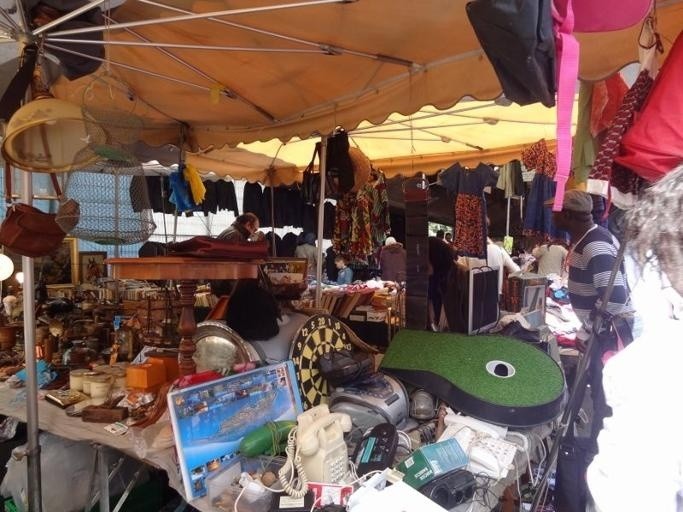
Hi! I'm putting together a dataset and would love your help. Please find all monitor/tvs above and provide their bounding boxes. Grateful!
[466,264,500,337]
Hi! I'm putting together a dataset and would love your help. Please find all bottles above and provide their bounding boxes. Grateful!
[68,364,125,402]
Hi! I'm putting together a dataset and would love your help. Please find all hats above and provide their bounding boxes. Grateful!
[542,189,593,213]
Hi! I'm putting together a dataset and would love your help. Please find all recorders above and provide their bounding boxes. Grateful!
[349,422,399,479]
[328,370,410,443]
[418,467,477,511]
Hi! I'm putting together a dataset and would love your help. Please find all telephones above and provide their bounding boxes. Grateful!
[435,422,518,479]
[295,403,352,483]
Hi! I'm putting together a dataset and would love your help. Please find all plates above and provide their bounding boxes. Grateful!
[175,321,254,373]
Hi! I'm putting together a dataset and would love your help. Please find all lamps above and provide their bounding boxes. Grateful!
[0,33,110,174]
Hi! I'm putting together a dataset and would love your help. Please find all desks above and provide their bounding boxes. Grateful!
[0,343,577,512]
[103,255,263,430]
[337,310,401,348]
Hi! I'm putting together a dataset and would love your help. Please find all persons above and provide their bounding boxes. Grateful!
[445,233,452,243]
[211,213,260,242]
[294,231,326,275]
[334,256,354,286]
[436,230,444,240]
[380,237,407,283]
[532,241,568,276]
[542,190,636,454]
[585,166,683,512]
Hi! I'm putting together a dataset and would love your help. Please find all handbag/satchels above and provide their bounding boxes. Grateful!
[403,187,428,202]
[176,236,273,262]
[0,198,80,257]
[466,0,556,107]
[302,172,343,206]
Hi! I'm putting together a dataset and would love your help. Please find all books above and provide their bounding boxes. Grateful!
[309,289,376,321]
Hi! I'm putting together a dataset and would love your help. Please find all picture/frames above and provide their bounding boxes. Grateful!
[263,255,308,286]
[78,250,107,286]
[0,234,78,291]
